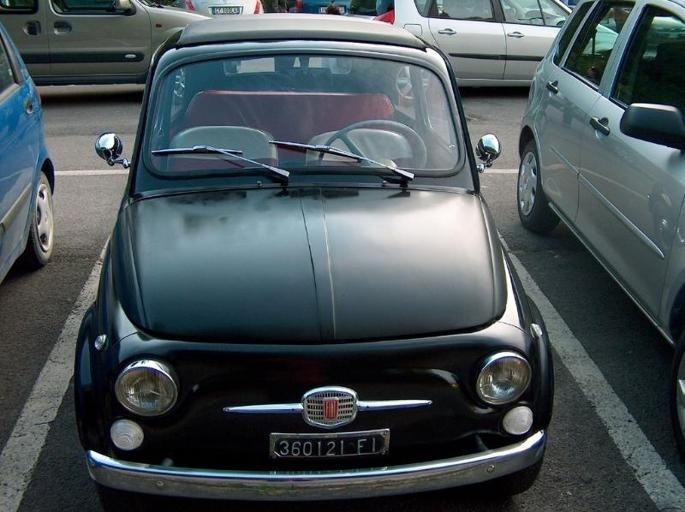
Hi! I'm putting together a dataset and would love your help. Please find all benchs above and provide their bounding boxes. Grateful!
[185,90,395,158]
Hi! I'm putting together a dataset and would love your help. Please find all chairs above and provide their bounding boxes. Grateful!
[306,128,413,168]
[168,126,278,171]
[649,40,685,109]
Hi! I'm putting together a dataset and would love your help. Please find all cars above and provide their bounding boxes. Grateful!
[515,1,685,463]
[0,22,56,286]
[73,14,555,510]
[283,1,352,15]
